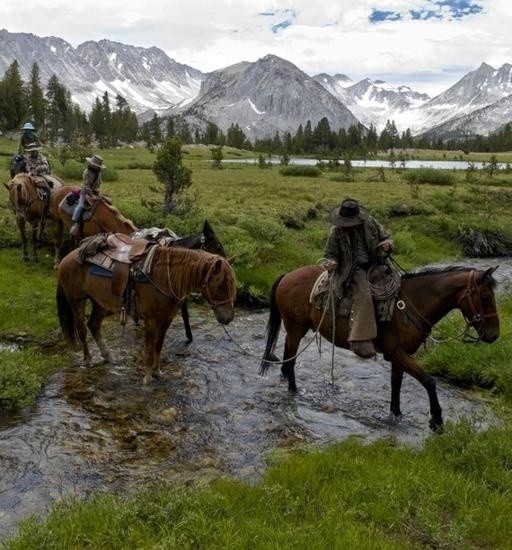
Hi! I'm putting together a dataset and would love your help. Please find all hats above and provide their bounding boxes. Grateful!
[86,155,106,169]
[21,123,35,130]
[24,143,43,152]
[331,198,369,227]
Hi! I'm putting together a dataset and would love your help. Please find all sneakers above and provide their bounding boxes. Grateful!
[69,225,80,236]
[351,340,376,358]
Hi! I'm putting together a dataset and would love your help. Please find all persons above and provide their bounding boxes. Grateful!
[24,142,51,177]
[17,122,44,157]
[322,199,396,358]
[70,154,106,234]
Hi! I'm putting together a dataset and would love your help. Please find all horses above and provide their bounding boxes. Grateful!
[50,183,138,270]
[4,173,65,264]
[129,219,225,344]
[9,151,52,179]
[55,245,238,392]
[259,259,499,438]
